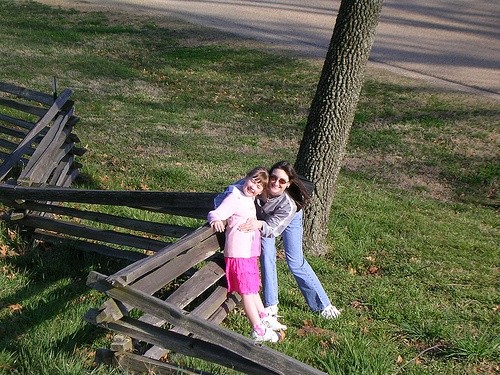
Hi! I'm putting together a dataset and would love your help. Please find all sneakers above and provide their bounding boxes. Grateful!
[252,327,283,343]
[264,304,279,323]
[320,306,342,318]
[260,315,288,331]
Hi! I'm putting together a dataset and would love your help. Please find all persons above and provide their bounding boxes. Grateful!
[208,167,281,342]
[238,161,340,319]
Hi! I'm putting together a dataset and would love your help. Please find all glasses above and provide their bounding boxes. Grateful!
[270,175,289,184]
